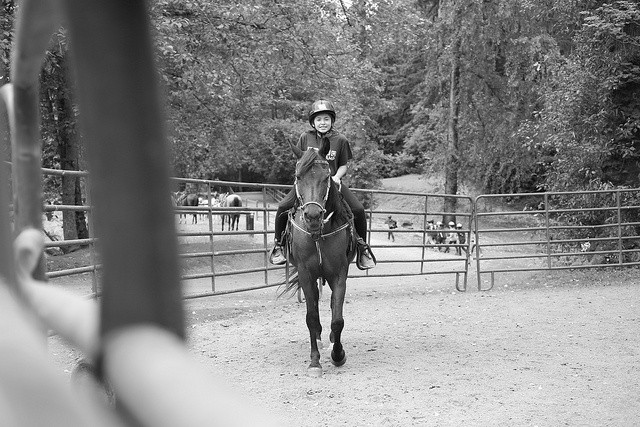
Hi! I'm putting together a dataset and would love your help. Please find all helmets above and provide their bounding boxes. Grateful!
[448,222,454,226]
[457,224,462,228]
[308,100,336,119]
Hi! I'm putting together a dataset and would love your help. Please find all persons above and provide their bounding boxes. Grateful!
[425,219,484,257]
[270,99,377,269]
[385,216,397,241]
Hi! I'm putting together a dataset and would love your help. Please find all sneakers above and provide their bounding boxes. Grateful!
[269,248,287,265]
[360,248,375,268]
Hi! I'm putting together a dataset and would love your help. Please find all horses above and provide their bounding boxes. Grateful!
[173,191,200,224]
[277,135,357,381]
[220,192,243,231]
